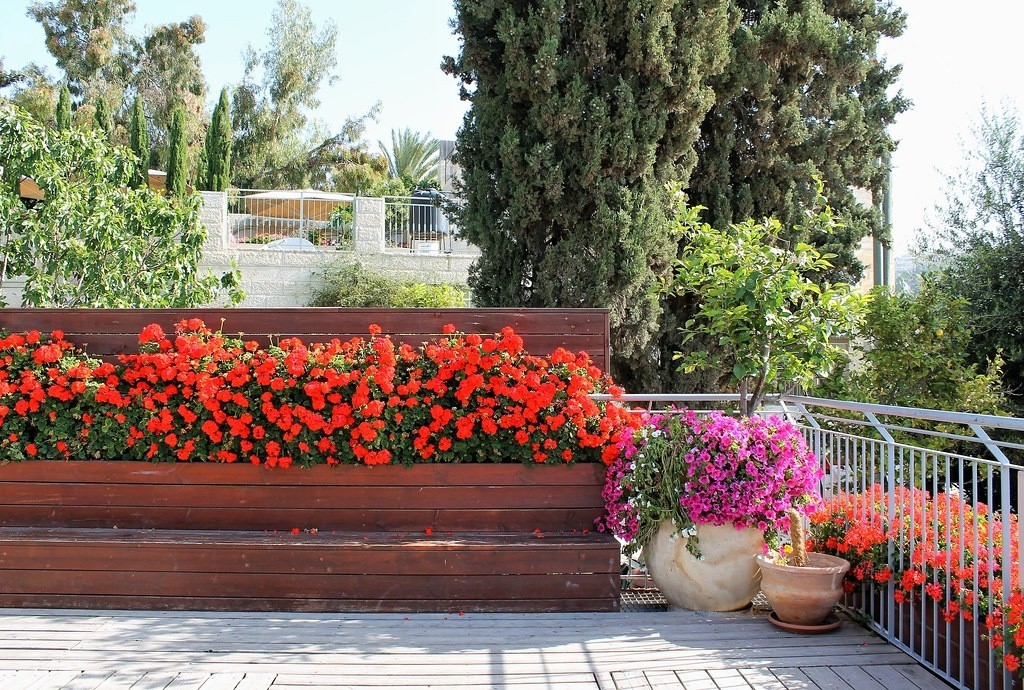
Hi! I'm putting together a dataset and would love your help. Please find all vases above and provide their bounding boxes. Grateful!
[641,517,768,612]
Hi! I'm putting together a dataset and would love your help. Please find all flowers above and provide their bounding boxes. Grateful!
[805,482,1024,690]
[235,233,288,244]
[306,230,336,246]
[0,315,648,466]
[591,403,826,593]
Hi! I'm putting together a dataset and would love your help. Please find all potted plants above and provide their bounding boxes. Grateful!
[756,507,852,624]
